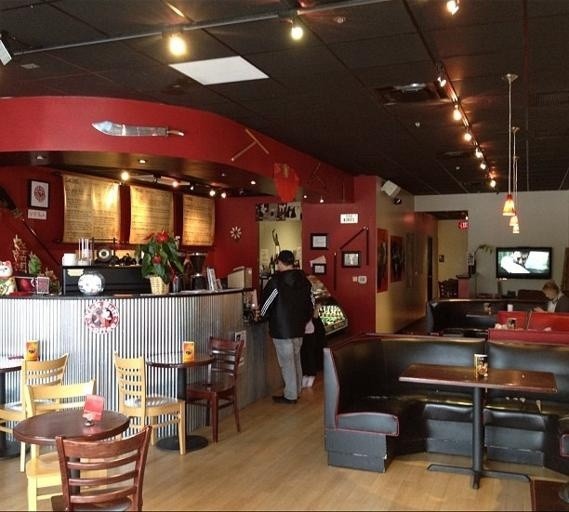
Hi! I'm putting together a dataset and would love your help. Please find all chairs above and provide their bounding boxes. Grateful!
[0,353,69,472]
[186,337,244,444]
[111,351,187,455]
[49,424,153,511]
[25,380,111,511]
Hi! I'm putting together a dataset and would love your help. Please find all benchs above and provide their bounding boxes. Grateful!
[427,290,568,336]
[323,333,568,473]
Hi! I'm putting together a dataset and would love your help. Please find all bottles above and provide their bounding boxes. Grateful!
[78,271,106,297]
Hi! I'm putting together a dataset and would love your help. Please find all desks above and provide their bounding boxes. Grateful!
[398,363,558,487]
[530,479,569,511]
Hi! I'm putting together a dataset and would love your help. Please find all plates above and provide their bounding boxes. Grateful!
[96,246,112,262]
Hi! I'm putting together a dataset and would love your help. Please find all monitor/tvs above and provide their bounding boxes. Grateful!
[495,247,553,279]
[61,265,152,294]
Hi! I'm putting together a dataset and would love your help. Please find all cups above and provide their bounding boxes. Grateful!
[507,304,513,312]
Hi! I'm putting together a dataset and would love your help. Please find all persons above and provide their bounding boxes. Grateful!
[512,249,529,268]
[533,280,569,312]
[260,251,313,403]
[301,295,321,388]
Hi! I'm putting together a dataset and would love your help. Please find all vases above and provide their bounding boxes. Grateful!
[148,276,170,295]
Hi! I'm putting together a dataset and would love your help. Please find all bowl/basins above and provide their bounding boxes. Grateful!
[62,252,89,266]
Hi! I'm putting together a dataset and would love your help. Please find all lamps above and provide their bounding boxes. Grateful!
[500,74,521,233]
[433,63,497,190]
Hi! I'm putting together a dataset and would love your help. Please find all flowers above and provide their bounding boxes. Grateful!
[136,230,185,283]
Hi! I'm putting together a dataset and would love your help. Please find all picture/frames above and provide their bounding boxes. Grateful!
[311,232,327,248]
[313,263,326,274]
[340,251,361,268]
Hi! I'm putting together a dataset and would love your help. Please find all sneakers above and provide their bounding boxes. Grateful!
[271,376,315,404]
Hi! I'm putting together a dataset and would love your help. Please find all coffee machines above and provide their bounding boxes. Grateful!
[183,250,208,290]
